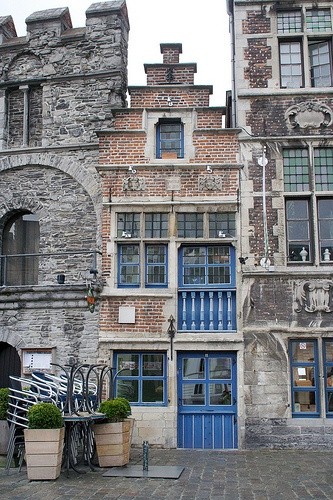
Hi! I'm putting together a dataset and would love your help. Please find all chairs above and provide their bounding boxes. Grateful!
[6,363,131,483]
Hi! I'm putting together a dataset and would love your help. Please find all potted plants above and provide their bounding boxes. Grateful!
[113,398,134,446]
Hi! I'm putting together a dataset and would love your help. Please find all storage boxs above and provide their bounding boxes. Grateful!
[0,388,15,455]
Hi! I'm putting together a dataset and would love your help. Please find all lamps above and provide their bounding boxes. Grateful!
[300,247,308,262]
[323,248,331,261]
